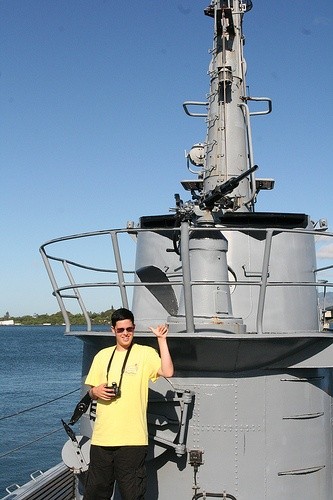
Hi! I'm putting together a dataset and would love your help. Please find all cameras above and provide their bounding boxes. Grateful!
[105,382,121,398]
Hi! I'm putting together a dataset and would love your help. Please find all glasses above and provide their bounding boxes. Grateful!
[115,327,134,333]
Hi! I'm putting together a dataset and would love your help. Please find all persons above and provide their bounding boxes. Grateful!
[83,307,174,500]
